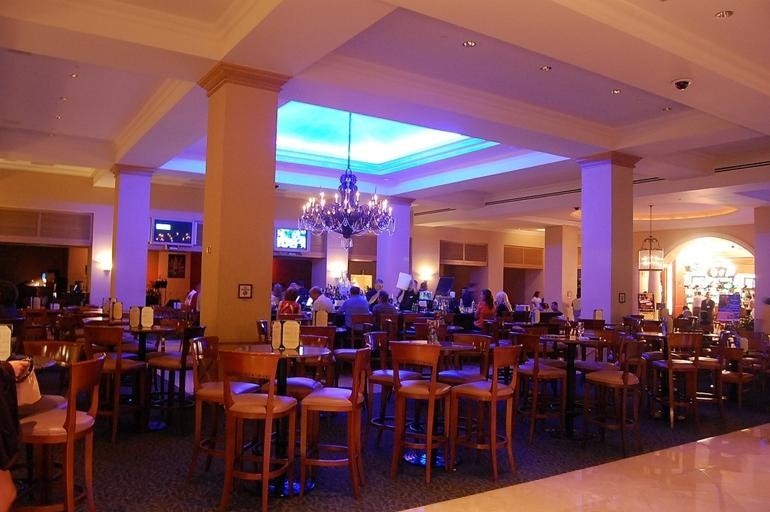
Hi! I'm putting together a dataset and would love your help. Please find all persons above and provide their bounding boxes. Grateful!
[571,293,581,322]
[676,306,691,319]
[700,293,714,312]
[0,281,30,512]
[529,290,565,319]
[395,280,433,311]
[271,279,397,336]
[185,279,200,313]
[447,288,513,335]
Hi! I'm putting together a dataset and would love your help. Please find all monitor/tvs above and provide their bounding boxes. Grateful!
[150,216,195,247]
[273,228,311,252]
[305,296,314,307]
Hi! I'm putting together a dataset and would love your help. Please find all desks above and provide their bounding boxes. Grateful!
[214,343,334,386]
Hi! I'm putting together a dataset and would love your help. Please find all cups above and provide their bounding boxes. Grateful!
[577,322,585,338]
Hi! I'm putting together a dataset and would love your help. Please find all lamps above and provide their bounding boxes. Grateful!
[299,101,396,247]
[637,204,664,272]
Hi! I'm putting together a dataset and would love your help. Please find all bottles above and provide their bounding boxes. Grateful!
[426,321,438,345]
[564,317,571,336]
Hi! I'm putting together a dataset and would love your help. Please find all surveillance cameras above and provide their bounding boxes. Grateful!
[574,207,579,210]
[673,79,691,91]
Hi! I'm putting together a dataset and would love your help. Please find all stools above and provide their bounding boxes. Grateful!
[219,349,298,511]
[272,284,770,443]
[190,335,261,477]
[19,353,108,512]
[298,344,371,498]
[1,284,200,443]
[389,340,455,486]
[450,344,520,484]
[363,331,422,454]
